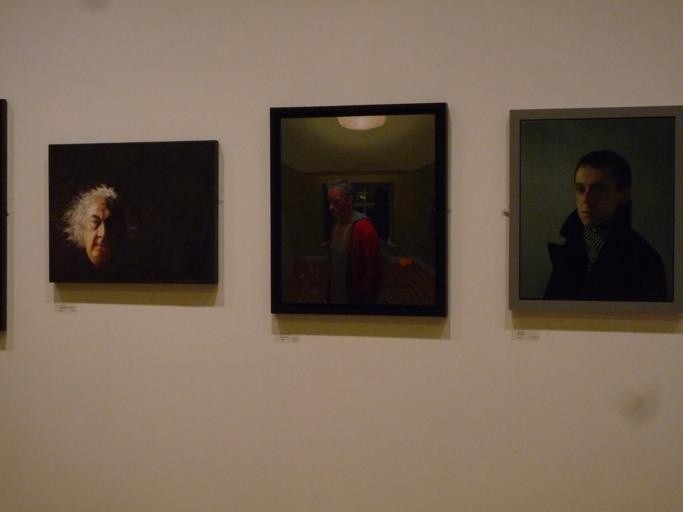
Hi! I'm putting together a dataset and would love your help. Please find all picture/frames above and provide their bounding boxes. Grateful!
[505,103,683,316]
[268,100,451,321]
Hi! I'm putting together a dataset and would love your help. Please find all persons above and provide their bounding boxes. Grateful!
[540,151,667,302]
[51,183,125,281]
[324,177,382,305]
